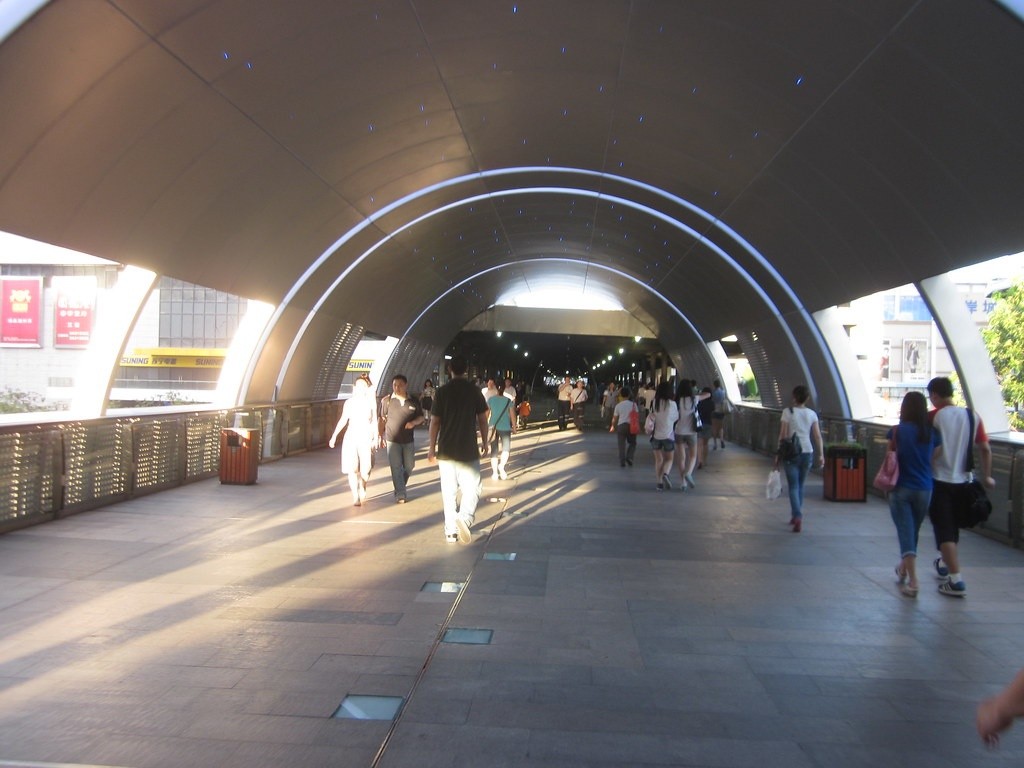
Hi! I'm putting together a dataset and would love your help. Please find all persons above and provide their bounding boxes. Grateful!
[774,385,825,533]
[973,665,1024,750]
[674,379,711,488]
[609,388,639,467]
[427,357,489,544]
[472,378,516,433]
[927,377,996,596]
[557,375,574,430]
[570,380,588,430]
[906,342,920,373]
[885,391,943,597]
[378,374,425,503]
[599,379,657,430]
[418,379,436,426]
[487,380,517,480]
[691,379,725,470]
[516,397,531,430]
[649,381,680,491]
[328,376,379,506]
[881,348,889,378]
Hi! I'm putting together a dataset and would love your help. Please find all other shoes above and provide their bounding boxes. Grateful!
[395,496,408,504]
[490,473,500,480]
[497,464,507,480]
[680,483,688,492]
[790,516,801,532]
[713,445,717,450]
[359,481,367,497]
[685,471,695,488]
[662,473,673,489]
[353,497,362,506]
[721,440,726,449]
[656,483,664,491]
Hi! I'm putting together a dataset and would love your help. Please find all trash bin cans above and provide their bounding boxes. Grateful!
[219,428,261,485]
[823,445,868,502]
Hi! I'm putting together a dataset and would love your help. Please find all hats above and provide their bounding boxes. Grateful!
[360,373,372,387]
[451,352,468,369]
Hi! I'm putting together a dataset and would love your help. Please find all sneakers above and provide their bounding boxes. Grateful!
[901,584,918,598]
[895,565,907,580]
[937,576,967,595]
[933,557,949,580]
[444,525,457,543]
[455,514,471,544]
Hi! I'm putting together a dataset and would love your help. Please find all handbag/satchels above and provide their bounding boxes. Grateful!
[722,399,732,414]
[872,426,900,498]
[950,479,992,528]
[630,402,641,435]
[644,413,656,435]
[766,470,783,500]
[486,425,496,444]
[694,407,704,432]
[774,431,803,460]
